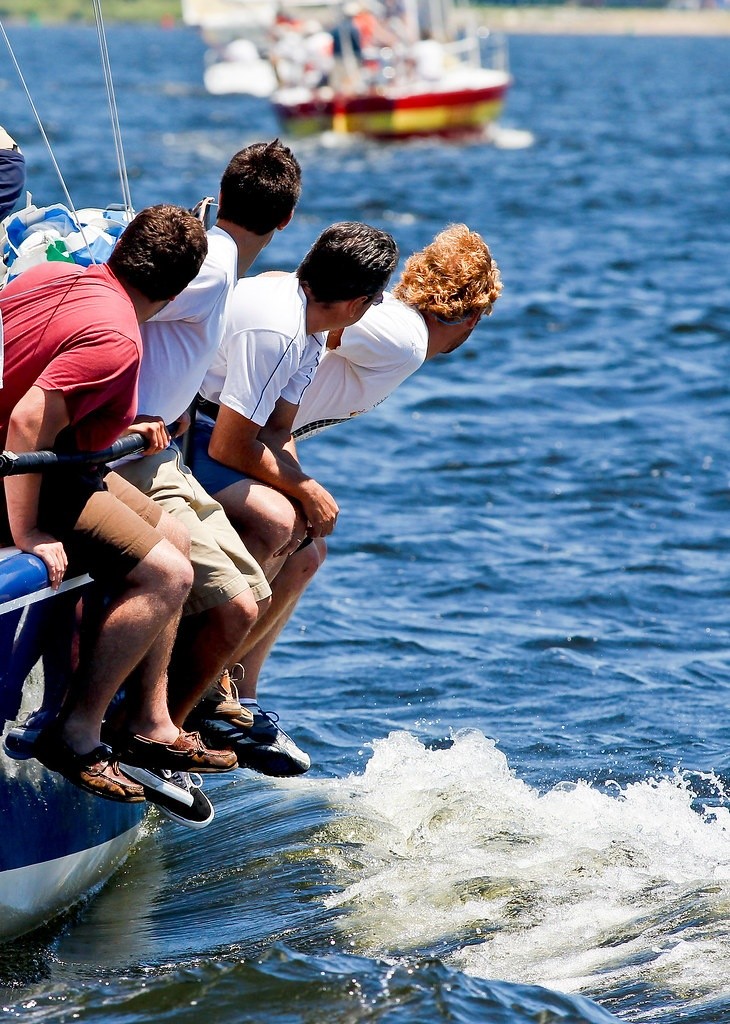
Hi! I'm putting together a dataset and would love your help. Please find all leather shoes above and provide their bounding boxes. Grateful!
[112,725,240,773]
[31,723,147,804]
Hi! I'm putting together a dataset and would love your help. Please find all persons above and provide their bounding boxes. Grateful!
[266,1,445,98]
[0,127,502,807]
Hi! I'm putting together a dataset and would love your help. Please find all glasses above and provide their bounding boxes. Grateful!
[367,293,384,306]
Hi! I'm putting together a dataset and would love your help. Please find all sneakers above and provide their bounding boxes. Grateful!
[194,662,254,727]
[2,706,57,762]
[200,703,311,778]
[117,759,215,829]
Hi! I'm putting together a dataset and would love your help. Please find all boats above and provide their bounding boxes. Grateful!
[0,433,191,948]
[272,0,512,146]
